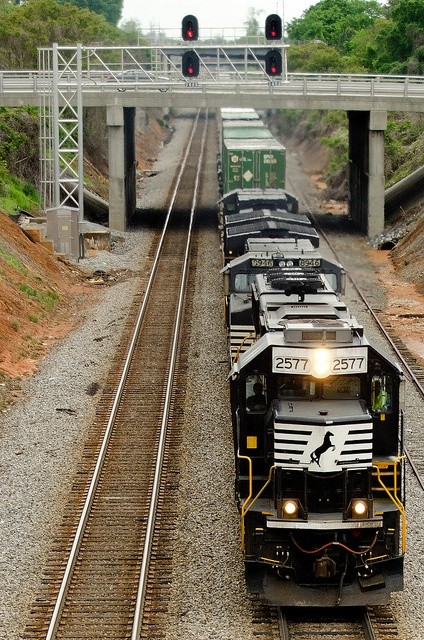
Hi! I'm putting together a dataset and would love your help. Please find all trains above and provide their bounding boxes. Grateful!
[216,106,408,610]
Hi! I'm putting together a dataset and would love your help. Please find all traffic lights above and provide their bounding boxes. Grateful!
[264,50,282,75]
[182,51,199,76]
[181,15,198,41]
[265,14,282,41]
